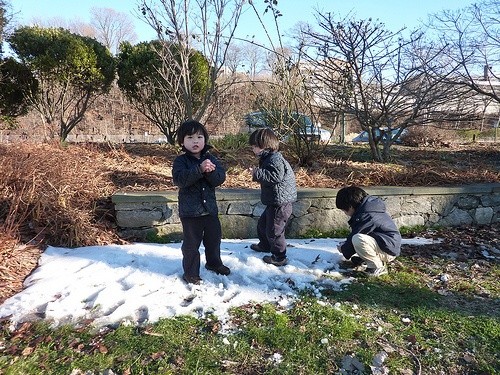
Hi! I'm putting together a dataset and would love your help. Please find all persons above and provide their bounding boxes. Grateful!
[172,121,230,284]
[249,129,297,267]
[335,184,402,278]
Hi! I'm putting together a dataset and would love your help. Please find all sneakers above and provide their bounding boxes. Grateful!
[263,255,287,266]
[351,256,367,268]
[182,272,202,291]
[205,261,230,276]
[363,266,388,277]
[250,244,271,253]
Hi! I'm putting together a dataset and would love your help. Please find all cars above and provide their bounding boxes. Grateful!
[352,126,418,147]
[238,110,331,144]
